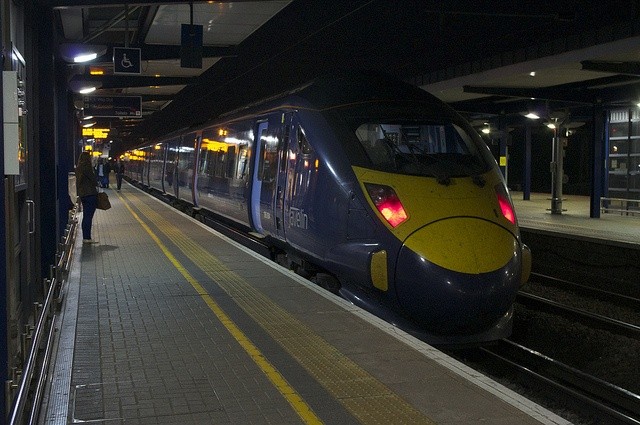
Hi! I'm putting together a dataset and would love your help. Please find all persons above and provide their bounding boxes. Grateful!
[91,155,111,188]
[75,151,101,244]
[114,156,125,193]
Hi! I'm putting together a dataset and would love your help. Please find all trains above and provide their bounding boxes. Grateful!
[113,68,532,345]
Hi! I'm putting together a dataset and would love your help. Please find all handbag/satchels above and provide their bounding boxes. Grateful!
[96,187,111,210]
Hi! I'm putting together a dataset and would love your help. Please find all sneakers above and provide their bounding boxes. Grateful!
[83,239,99,244]
